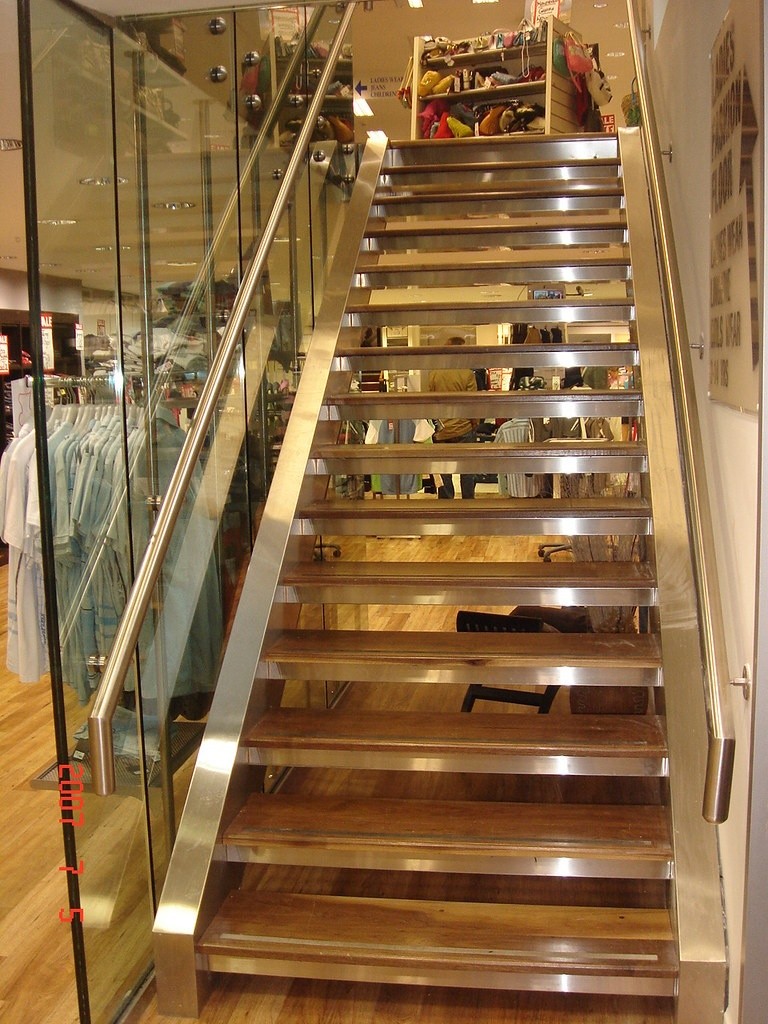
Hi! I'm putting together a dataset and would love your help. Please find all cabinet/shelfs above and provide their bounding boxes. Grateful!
[0,309,82,459]
[255,33,354,147]
[411,14,582,140]
[246,352,307,495]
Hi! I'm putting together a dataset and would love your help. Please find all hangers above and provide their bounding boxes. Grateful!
[4,375,144,456]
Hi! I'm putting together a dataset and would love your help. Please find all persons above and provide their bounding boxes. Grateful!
[428,336,480,499]
